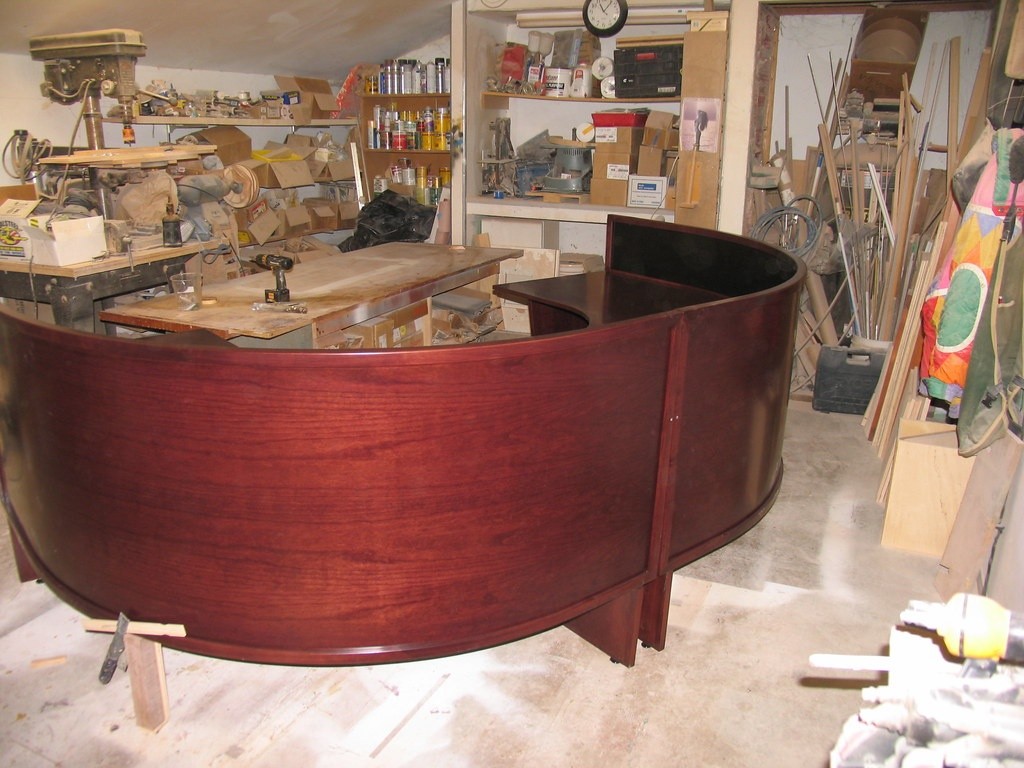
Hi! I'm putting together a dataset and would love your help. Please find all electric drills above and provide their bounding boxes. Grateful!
[250,255,295,303]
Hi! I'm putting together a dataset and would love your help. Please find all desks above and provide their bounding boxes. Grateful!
[99,240,521,355]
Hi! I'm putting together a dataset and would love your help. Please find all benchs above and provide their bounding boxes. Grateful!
[0,214,810,734]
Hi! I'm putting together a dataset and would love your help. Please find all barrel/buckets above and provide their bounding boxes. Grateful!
[545,67,572,98]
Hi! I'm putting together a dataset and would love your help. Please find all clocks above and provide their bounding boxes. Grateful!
[592,56,615,79]
[577,1,627,37]
[600,77,616,99]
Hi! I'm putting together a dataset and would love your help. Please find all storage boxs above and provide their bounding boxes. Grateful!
[0,75,417,276]
[346,296,448,351]
[555,10,728,212]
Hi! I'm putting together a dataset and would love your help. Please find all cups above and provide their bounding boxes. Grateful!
[170,273,203,310]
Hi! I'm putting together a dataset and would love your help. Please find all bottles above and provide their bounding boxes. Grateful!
[363,58,451,205]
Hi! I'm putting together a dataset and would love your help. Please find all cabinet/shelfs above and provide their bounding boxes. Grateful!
[356,91,450,208]
[448,0,780,265]
